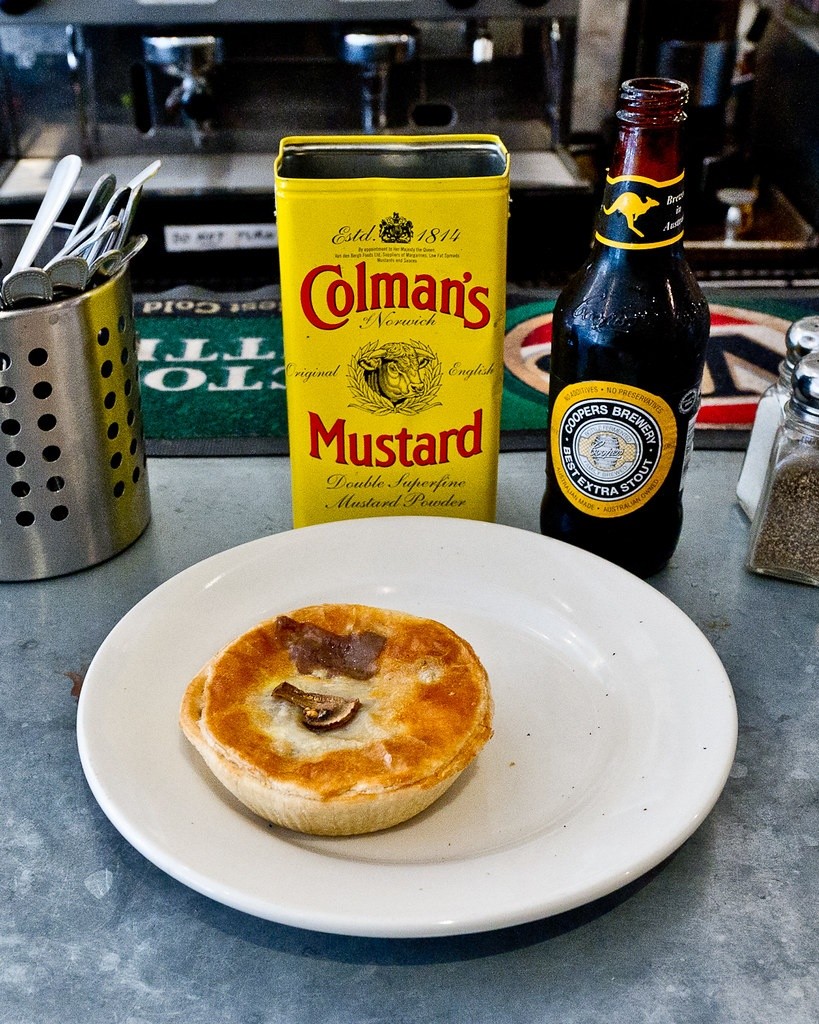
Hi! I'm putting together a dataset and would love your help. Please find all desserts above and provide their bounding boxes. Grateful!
[178,603,497,837]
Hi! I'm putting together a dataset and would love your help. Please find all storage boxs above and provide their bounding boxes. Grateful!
[274,134,513,529]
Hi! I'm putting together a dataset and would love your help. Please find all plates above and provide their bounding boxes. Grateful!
[74,516,741,939]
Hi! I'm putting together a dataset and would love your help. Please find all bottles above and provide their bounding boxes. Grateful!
[737,316,819,521]
[745,349,819,587]
[539,77,711,578]
